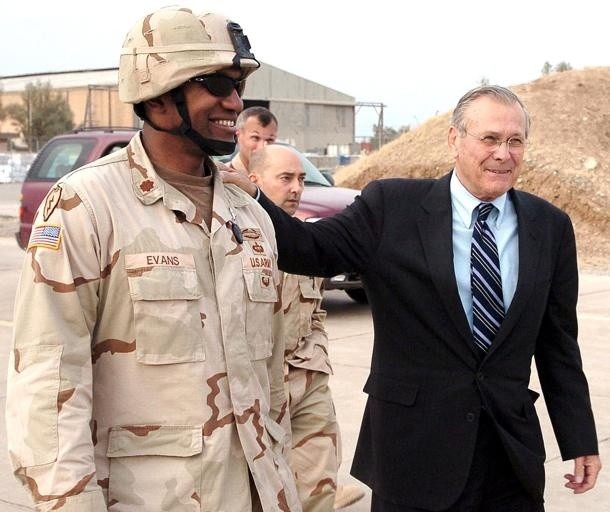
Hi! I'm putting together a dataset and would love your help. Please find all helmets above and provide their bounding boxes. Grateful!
[118,5,261,104]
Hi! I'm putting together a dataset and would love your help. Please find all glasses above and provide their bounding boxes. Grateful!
[189,70,247,98]
[465,129,525,149]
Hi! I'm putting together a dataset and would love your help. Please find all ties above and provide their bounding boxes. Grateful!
[470,202,506,353]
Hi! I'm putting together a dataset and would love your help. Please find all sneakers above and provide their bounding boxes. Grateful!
[332,483,368,512]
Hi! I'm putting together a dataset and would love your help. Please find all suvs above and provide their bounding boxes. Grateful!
[17,123,386,305]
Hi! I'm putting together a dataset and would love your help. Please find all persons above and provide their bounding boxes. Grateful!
[213,84,603,511]
[248,143,371,511]
[8,8,346,512]
[324,141,351,167]
[224,106,279,182]
